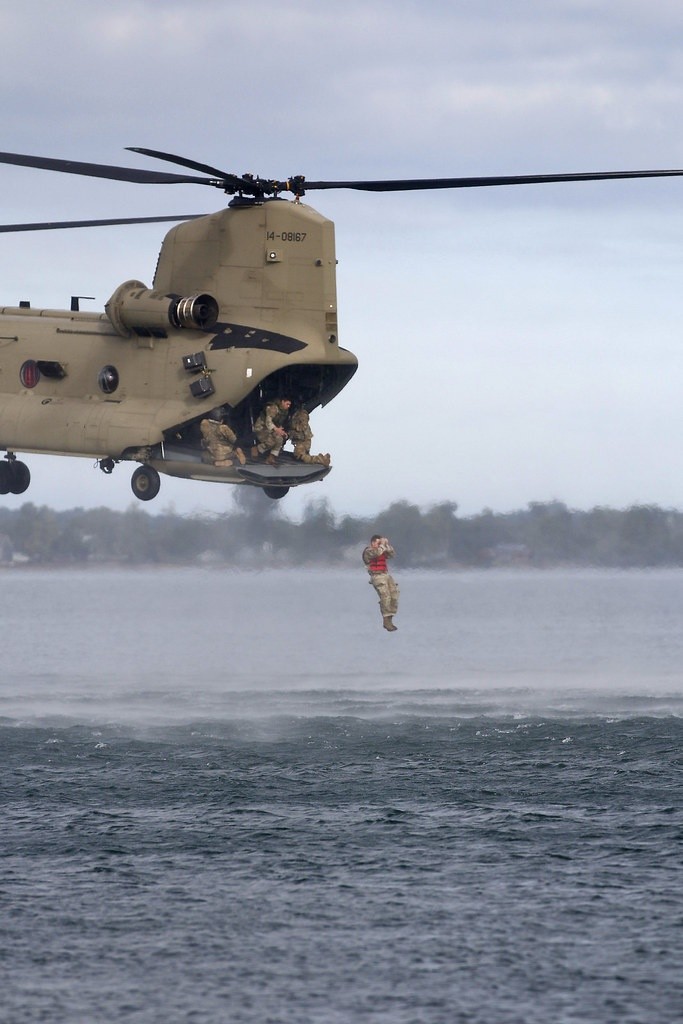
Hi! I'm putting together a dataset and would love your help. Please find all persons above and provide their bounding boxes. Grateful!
[362,535,400,633]
[201,395,329,465]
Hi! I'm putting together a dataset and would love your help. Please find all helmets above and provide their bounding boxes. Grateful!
[209,407,231,422]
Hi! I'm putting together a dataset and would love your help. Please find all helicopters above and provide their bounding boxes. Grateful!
[0,143,682,502]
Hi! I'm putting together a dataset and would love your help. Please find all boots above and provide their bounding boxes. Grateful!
[264,453,281,467]
[383,615,397,632]
[214,459,234,467]
[318,453,330,468]
[250,445,260,463]
[235,447,246,465]
